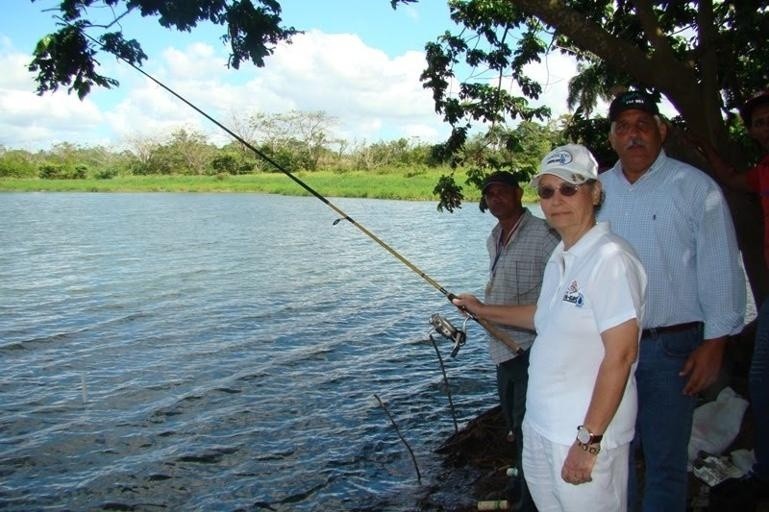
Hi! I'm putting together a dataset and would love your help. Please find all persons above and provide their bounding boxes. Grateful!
[450,143,651,512]
[480,170,562,511]
[594,91,749,511]
[676,90,769,511]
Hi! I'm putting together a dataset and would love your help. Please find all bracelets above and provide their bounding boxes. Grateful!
[575,438,602,455]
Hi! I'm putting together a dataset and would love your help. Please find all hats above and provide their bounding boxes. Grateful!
[535,145,599,186]
[609,91,658,120]
[480,170,517,193]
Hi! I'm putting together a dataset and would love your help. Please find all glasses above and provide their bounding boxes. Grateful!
[537,182,587,199]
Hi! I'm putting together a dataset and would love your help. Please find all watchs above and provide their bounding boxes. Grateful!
[576,424,604,446]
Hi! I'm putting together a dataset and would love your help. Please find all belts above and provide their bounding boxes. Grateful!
[644,323,700,338]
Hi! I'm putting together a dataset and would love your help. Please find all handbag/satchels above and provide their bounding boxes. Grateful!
[689,388,748,471]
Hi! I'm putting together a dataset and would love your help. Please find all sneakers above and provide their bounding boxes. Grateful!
[693,449,741,486]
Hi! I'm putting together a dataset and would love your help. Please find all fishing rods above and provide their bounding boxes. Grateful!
[48,14,525,357]
